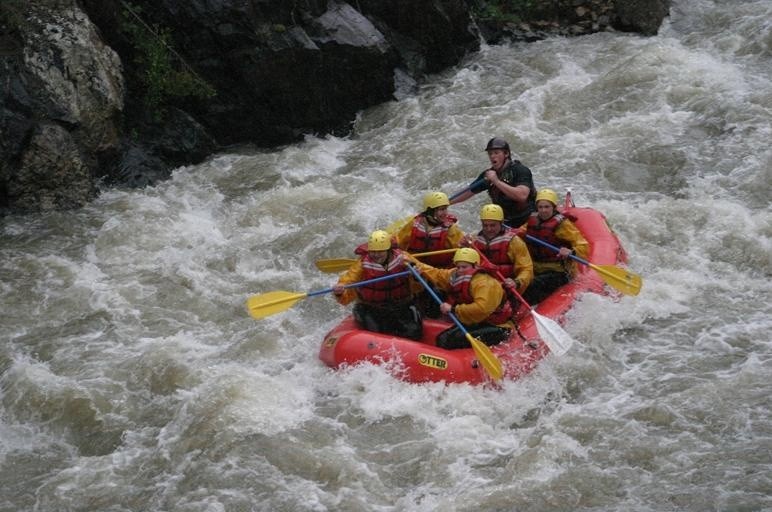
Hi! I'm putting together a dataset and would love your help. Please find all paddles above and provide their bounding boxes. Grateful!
[315,247,460,273]
[248,269,411,320]
[501,222,642,297]
[467,237,573,357]
[373,171,492,238]
[402,261,505,381]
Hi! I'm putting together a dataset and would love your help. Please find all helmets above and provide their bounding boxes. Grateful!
[453,247,480,264]
[534,189,560,206]
[485,137,509,150]
[422,193,449,212]
[479,203,503,219]
[367,229,393,253]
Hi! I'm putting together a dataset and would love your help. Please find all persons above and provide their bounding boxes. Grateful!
[468,204,533,320]
[401,248,514,350]
[446,137,537,242]
[333,230,422,342]
[395,192,467,318]
[521,188,590,308]
